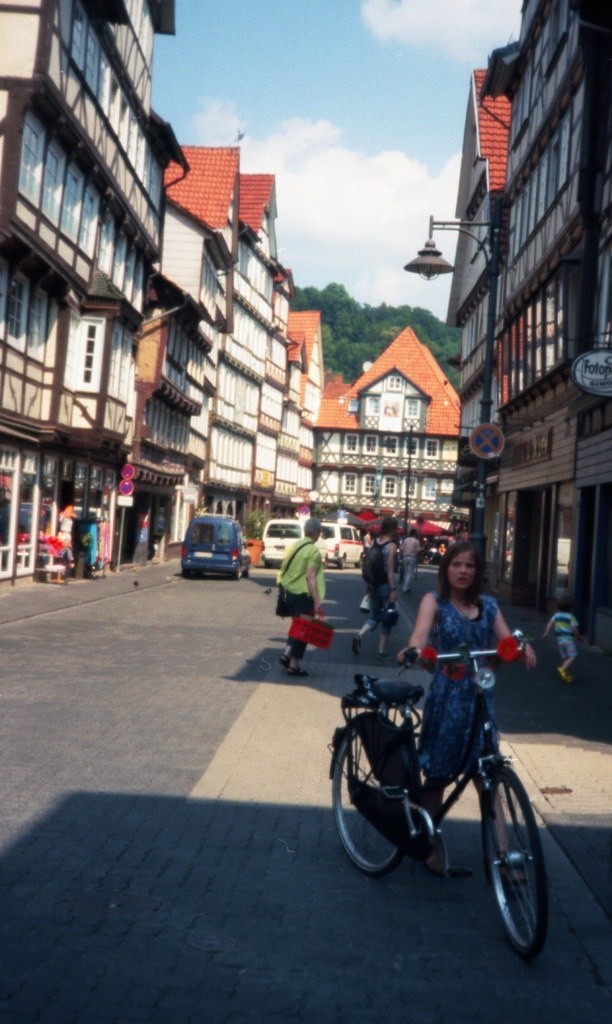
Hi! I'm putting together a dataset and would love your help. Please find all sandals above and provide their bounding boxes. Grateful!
[279,655,289,667]
[288,666,308,676]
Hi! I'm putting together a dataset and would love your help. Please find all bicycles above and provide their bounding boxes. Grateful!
[324,627,548,964]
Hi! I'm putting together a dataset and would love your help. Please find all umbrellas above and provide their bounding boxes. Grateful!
[348,513,453,537]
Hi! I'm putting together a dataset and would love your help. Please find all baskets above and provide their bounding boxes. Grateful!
[288,610,336,651]
[381,600,400,627]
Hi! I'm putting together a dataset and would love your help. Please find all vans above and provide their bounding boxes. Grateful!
[180,516,253,580]
[321,523,367,568]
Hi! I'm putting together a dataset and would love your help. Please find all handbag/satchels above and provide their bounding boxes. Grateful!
[275,587,291,617]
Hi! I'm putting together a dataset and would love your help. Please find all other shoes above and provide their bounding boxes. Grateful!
[378,652,394,659]
[352,635,360,656]
[498,864,528,886]
[566,675,574,682]
[558,667,567,679]
[403,588,411,593]
[422,854,450,880]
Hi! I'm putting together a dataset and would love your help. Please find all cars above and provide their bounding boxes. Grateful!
[260,520,306,568]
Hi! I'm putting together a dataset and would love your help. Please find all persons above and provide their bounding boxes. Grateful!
[542,594,584,684]
[57,503,78,569]
[351,517,399,659]
[362,530,456,592]
[395,540,538,889]
[275,517,326,677]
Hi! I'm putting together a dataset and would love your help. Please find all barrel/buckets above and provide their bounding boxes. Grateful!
[380,600,400,625]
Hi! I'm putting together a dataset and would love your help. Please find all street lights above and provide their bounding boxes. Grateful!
[405,215,505,595]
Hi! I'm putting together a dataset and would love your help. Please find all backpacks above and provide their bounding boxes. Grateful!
[362,535,399,582]
